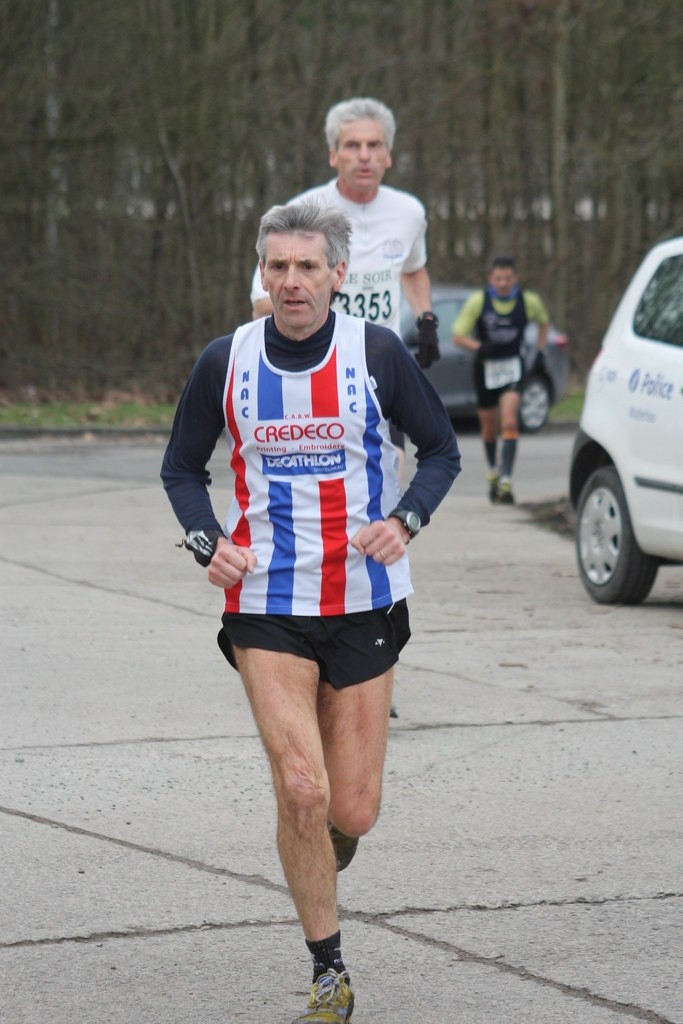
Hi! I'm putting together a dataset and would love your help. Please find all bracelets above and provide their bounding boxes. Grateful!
[422,312,438,321]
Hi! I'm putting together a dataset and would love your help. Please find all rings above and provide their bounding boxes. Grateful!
[379,550,386,558]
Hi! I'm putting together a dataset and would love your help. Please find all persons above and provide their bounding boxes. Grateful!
[160,205,461,1024]
[250,98,446,507]
[451,256,549,503]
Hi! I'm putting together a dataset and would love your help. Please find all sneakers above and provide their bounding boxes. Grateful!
[328,821,360,872]
[291,968,354,1024]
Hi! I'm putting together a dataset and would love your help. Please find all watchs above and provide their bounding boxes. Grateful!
[389,508,422,538]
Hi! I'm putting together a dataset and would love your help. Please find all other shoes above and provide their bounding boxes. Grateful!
[488,469,512,502]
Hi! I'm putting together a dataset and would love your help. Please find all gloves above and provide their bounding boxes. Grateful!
[411,313,441,368]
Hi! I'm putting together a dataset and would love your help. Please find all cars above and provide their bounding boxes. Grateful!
[400,285,569,434]
[569,239,683,605]
